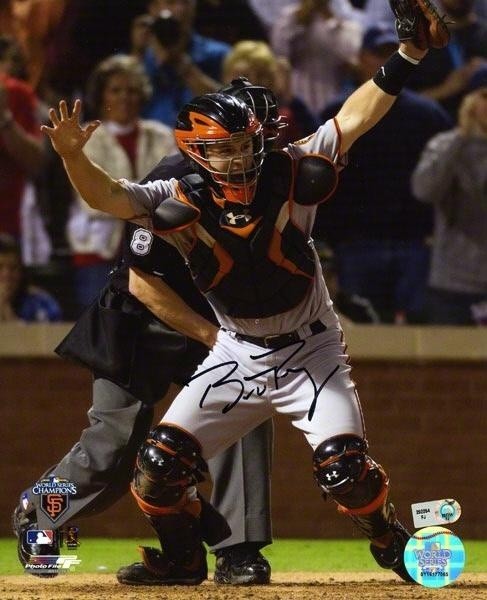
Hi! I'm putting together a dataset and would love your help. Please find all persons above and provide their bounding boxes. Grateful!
[11,76,291,584]
[39,0,453,585]
[0,0,487,326]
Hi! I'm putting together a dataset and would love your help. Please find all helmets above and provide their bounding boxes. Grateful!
[173,76,288,207]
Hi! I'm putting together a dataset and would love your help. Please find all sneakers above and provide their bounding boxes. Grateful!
[116,544,208,587]
[213,548,270,587]
[370,504,415,582]
[11,505,61,578]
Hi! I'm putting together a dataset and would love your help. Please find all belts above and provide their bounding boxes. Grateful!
[223,319,328,350]
